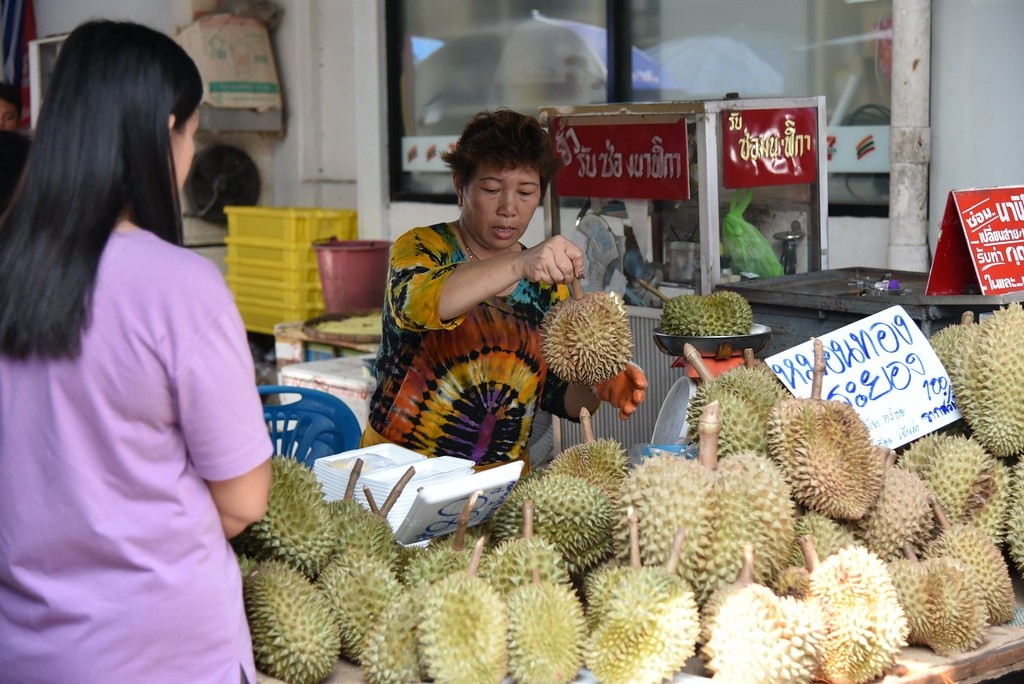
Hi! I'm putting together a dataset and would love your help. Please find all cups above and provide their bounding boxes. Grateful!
[669,242,700,283]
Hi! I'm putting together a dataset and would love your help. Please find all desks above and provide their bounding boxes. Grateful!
[272,323,380,357]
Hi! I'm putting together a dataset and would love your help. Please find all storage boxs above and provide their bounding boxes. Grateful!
[220,206,358,337]
[277,352,377,434]
[174,13,282,115]
[312,442,526,547]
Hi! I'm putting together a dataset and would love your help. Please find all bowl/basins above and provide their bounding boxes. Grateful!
[314,442,525,552]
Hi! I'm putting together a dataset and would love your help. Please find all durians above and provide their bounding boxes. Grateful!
[228,289,1024,684]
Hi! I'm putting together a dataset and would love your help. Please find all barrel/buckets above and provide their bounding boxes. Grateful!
[314,235,393,314]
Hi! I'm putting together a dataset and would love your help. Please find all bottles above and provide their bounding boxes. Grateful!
[623,224,645,281]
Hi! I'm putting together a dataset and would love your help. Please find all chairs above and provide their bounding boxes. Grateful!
[258,384,361,470]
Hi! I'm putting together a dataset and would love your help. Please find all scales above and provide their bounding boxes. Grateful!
[649,324,773,446]
